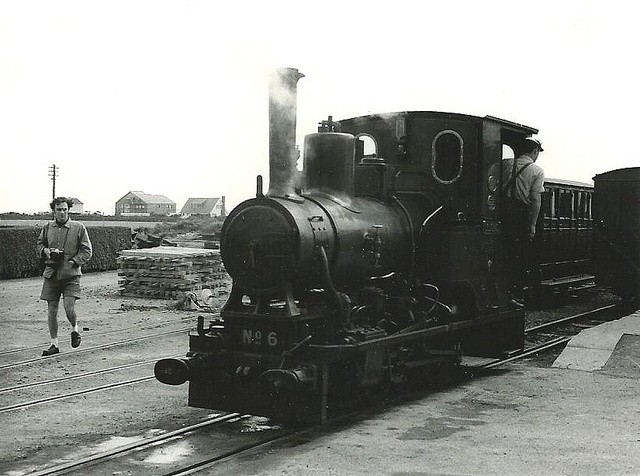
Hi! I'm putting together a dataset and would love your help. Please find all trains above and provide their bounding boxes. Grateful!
[154,68,539,425]
[592,167,640,309]
[523,177,594,279]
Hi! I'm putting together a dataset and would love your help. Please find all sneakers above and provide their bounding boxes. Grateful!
[506,298,524,309]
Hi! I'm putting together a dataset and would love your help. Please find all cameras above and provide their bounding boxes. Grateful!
[50,250,65,260]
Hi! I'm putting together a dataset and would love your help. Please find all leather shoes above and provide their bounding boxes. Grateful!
[43,345,59,355]
[71,332,81,348]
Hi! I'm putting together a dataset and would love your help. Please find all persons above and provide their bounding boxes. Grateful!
[35,196,93,356]
[501,134,544,293]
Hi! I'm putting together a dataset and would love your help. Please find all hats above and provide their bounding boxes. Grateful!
[525,135,543,152]
